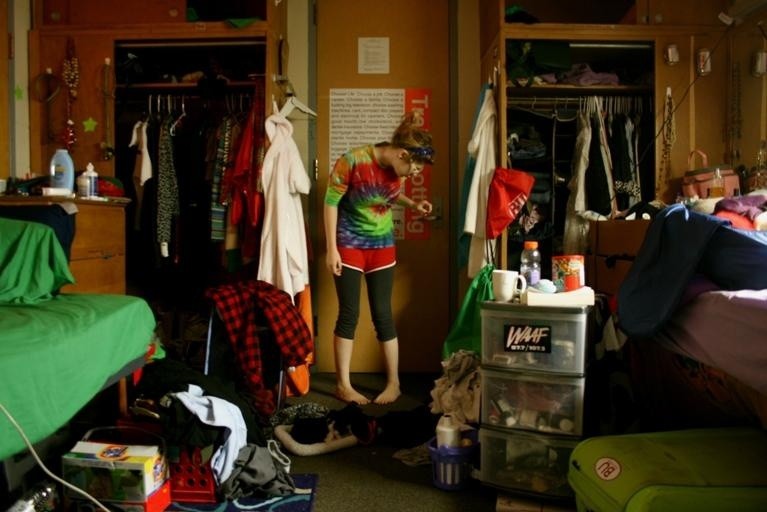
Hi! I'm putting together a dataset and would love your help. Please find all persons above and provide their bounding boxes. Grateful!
[322,110,434,406]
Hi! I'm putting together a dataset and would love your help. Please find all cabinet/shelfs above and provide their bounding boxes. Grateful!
[475,300,589,505]
[2,197,130,417]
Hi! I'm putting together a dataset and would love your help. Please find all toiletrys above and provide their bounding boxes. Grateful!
[50,148,75,192]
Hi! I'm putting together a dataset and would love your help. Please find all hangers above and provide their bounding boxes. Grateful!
[277,80,320,122]
[168,94,205,137]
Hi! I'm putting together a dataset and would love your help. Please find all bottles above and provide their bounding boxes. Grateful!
[82,163,98,197]
[709,168,725,197]
[521,241,541,283]
[490,392,515,430]
[549,412,573,430]
[7,485,50,511]
[435,415,460,486]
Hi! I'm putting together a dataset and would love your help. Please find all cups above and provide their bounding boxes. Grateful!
[492,270,526,303]
[563,273,581,290]
